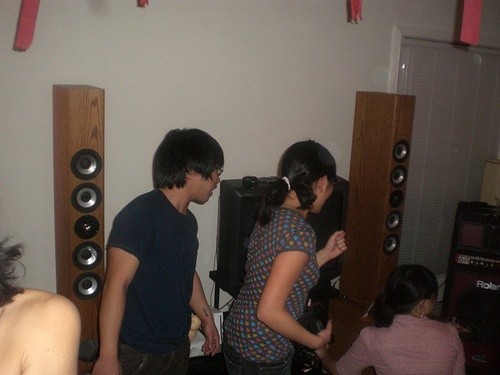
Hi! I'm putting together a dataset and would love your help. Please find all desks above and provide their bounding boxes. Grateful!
[208,271,342,375]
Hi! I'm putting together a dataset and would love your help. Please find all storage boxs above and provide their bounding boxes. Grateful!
[479,158,500,208]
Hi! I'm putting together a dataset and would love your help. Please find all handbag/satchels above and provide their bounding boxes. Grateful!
[289,308,336,350]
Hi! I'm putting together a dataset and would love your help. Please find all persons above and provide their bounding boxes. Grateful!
[0,235,81,375]
[222,140,348,375]
[316,264,465,374]
[92,128,224,375]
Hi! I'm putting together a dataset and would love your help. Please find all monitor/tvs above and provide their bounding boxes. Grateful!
[216,174,350,282]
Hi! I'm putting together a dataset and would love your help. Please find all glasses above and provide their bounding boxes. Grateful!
[213,166,224,177]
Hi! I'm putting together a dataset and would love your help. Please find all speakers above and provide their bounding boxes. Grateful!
[341,90,416,311]
[53,85,106,375]
[440,200,500,375]
